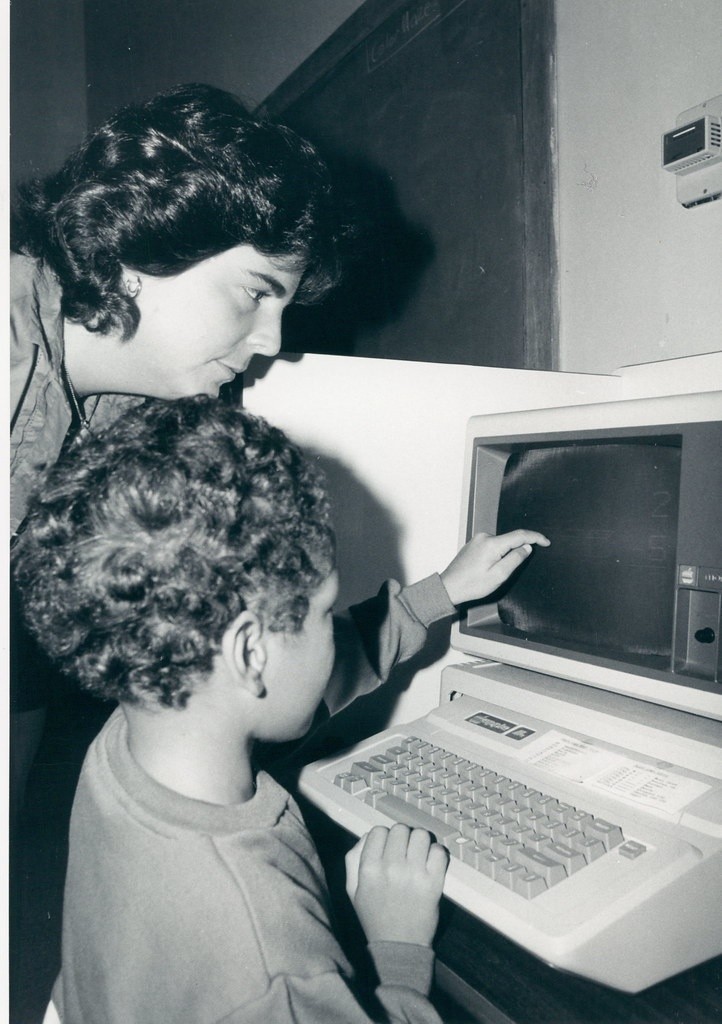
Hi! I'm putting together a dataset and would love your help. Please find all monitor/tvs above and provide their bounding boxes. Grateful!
[449,391,721,722]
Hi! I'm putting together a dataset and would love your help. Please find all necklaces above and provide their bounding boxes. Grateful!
[67,374,101,428]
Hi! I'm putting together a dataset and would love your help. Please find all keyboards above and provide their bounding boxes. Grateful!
[299,724,700,935]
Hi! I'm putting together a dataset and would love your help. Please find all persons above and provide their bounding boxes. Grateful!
[10,87,368,814]
[10,391,550,1024]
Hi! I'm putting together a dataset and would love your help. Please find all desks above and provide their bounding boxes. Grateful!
[329,834,722,1024]
